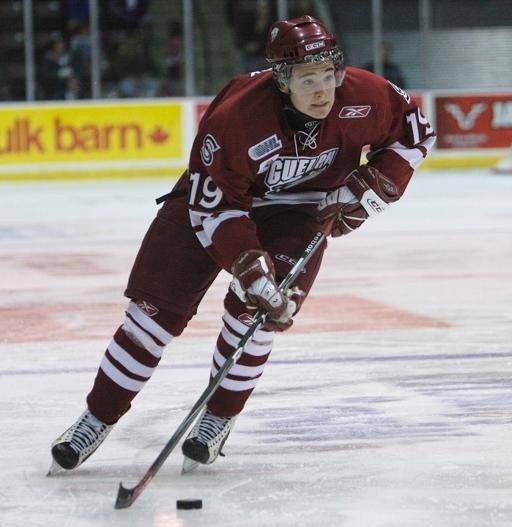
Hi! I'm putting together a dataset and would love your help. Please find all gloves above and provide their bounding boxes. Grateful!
[232,248,303,332]
[315,164,400,238]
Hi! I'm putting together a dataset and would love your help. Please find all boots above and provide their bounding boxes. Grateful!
[182,407,237,464]
[52,402,131,470]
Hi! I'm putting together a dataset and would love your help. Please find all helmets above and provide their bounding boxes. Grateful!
[265,15,346,95]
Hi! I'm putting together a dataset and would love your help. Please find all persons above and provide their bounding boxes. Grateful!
[0,1,187,100]
[363,40,405,90]
[52,16,438,471]
[223,1,344,76]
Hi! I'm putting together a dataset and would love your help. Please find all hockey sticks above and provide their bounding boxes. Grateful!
[113,216,334,511]
